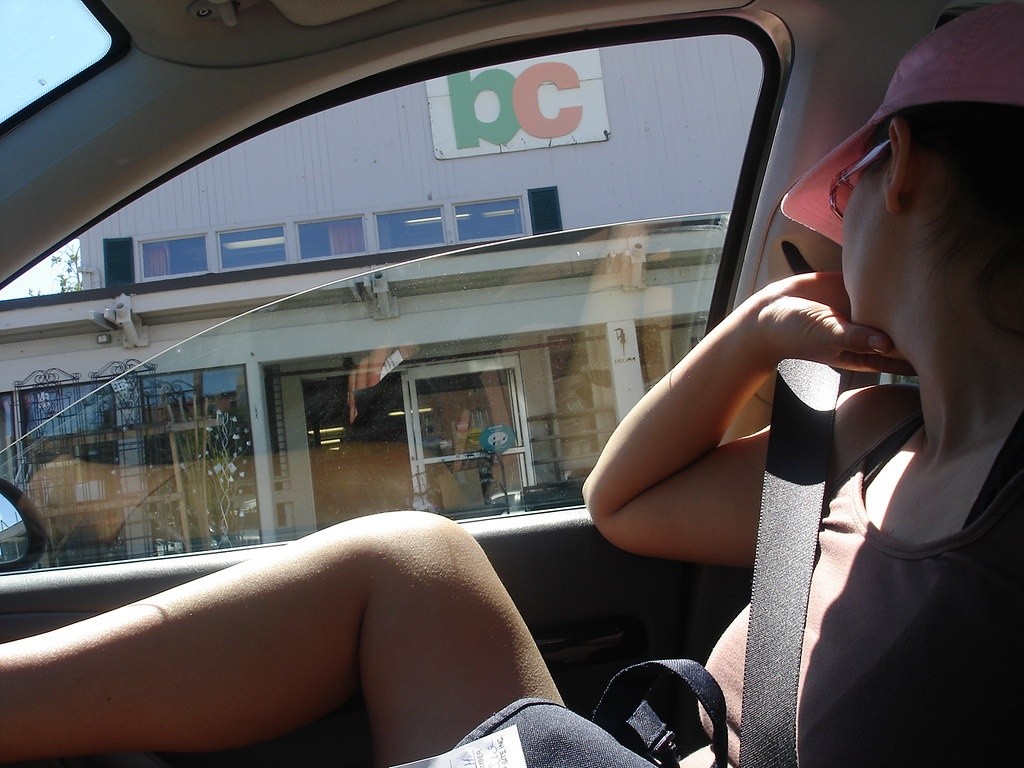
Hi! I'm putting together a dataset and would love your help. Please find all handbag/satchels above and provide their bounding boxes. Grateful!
[448,697,660,768]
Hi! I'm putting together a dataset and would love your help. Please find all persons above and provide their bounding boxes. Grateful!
[0,1,1024,768]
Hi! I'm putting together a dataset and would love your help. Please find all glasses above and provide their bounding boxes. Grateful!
[829,139,892,222]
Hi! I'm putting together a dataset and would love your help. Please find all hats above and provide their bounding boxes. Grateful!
[781,0,1024,248]
